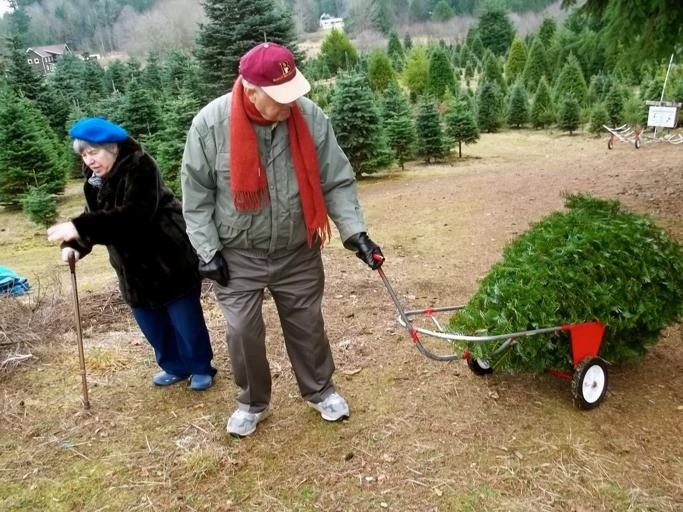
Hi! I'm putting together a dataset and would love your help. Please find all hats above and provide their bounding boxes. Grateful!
[240,41,312,105]
[68,116,128,144]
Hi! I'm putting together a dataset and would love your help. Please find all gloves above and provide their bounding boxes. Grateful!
[351,232,385,270]
[60,247,79,262]
[198,252,232,287]
[45,219,79,244]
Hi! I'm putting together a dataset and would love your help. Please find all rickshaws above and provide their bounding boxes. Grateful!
[372,253,608,410]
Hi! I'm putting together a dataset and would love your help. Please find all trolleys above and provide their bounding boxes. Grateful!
[602,124,645,149]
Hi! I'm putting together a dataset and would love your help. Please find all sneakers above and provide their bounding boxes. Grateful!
[307,391,350,421]
[190,373,213,390]
[226,408,272,437]
[152,370,183,386]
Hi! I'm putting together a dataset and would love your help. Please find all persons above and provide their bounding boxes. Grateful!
[46,118,218,391]
[180,41,385,438]
